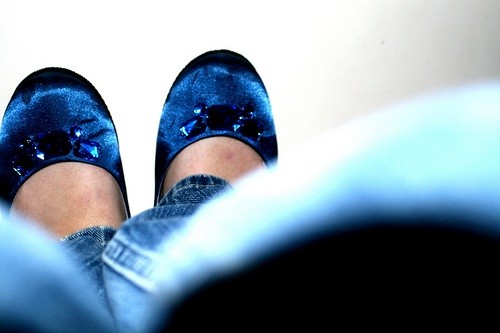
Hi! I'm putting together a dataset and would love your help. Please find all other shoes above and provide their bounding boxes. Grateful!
[155,49,279,208]
[0,66,130,231]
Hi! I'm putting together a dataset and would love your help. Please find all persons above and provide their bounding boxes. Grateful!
[0,48,500,332]
